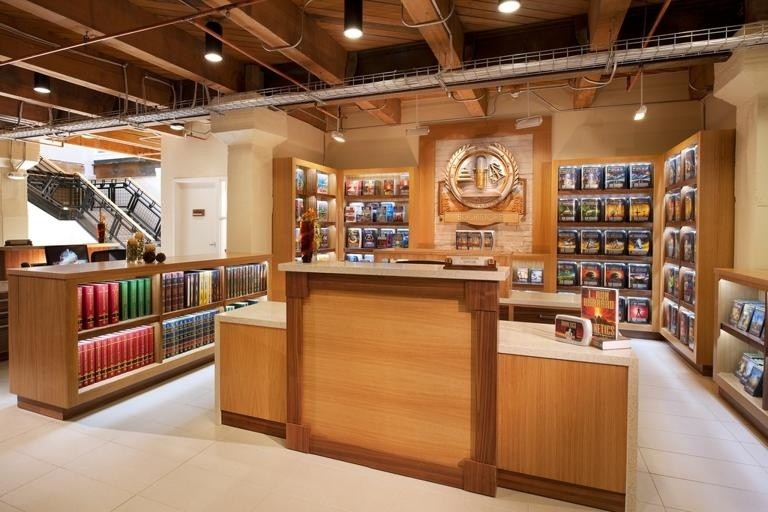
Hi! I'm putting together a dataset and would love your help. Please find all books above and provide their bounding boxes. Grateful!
[660,144,696,352]
[589,333,636,351]
[530,268,546,284]
[557,161,655,325]
[516,263,530,282]
[582,286,619,338]
[455,227,494,252]
[295,166,410,263]
[75,262,270,392]
[728,297,768,396]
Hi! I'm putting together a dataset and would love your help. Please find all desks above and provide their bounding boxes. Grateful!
[213,258,640,512]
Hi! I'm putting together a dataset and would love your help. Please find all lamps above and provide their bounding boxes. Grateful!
[497,0,521,15]
[203,17,223,62]
[633,73,647,122]
[329,107,349,146]
[512,85,543,130]
[344,0,363,39]
[33,72,50,95]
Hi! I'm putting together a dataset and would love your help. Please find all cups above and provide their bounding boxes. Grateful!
[127,238,137,262]
[134,233,144,263]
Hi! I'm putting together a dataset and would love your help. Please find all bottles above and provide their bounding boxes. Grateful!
[97,206,106,243]
